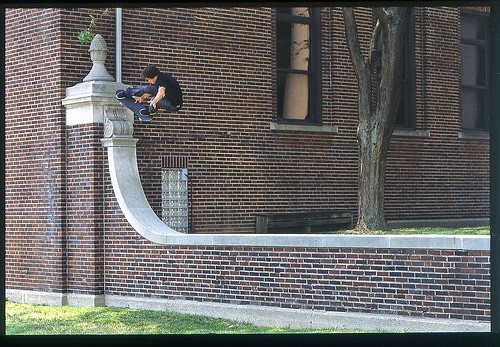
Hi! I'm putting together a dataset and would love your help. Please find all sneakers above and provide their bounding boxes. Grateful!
[116,90,126,99]
[139,108,158,117]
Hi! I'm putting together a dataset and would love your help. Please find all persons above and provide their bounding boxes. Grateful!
[115,65,183,117]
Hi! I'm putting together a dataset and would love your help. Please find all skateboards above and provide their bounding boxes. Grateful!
[115,89,154,122]
